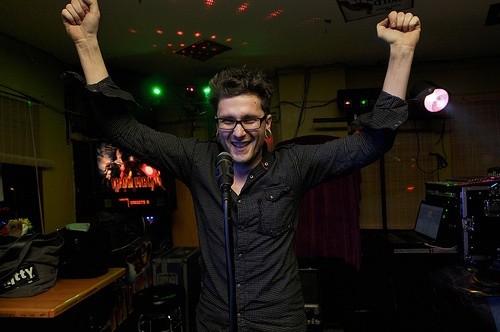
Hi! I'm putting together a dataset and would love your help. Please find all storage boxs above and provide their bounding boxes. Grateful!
[424,181,500,265]
[152,247,202,331]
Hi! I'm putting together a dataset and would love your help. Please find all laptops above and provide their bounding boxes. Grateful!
[389,200,445,243]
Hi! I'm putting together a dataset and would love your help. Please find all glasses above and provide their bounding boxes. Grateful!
[215,114,266,130]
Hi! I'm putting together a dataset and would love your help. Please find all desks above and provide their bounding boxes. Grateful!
[360,230,459,312]
[0,267,125,332]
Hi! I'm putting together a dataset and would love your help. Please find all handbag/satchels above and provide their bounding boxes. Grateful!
[0,228,65,298]
[32,222,114,278]
[86,209,145,255]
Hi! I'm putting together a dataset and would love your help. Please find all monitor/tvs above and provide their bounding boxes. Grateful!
[89,138,177,214]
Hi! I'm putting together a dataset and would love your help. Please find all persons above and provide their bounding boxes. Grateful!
[113,149,123,165]
[60,0,423,332]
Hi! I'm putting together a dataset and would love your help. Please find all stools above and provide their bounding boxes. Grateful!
[132,284,184,332]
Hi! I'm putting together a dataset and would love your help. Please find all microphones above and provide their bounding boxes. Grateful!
[429,152,440,156]
[215,152,234,191]
